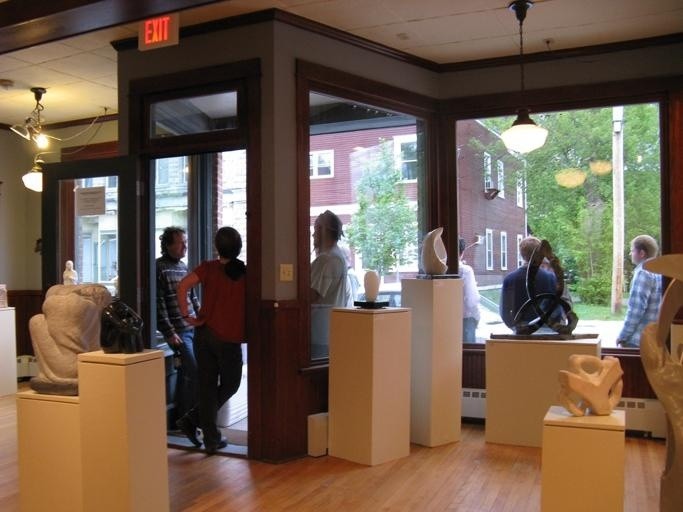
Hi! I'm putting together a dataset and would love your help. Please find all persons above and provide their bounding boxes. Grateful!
[459,239,480,344]
[615,234,662,350]
[309,210,347,359]
[155,225,201,432]
[61,260,79,287]
[498,237,572,334]
[174,227,246,450]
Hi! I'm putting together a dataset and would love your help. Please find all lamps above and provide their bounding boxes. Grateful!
[500,1,548,156]
[10,84,55,193]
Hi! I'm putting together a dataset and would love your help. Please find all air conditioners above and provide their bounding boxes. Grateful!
[474,235,484,245]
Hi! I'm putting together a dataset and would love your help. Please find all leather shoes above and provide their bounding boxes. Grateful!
[168,414,228,452]
